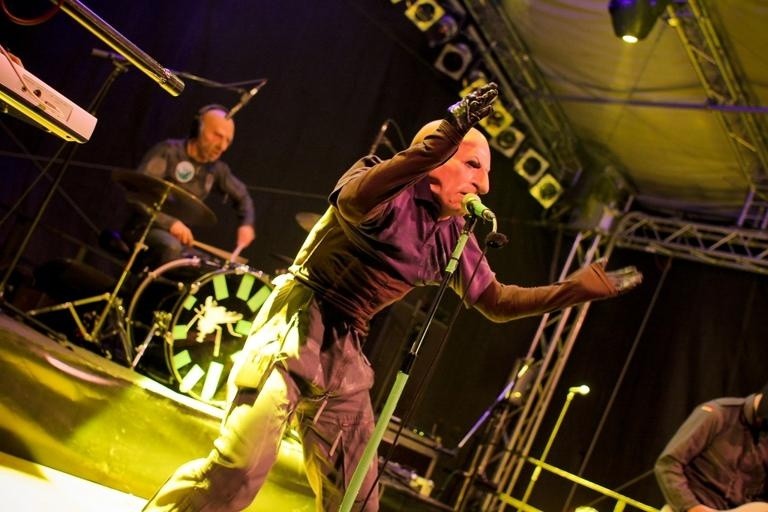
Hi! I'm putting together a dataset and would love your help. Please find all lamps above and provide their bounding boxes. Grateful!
[488,126,526,159]
[434,44,473,81]
[459,68,488,98]
[427,15,460,46]
[608,0,665,43]
[405,0,445,32]
[513,148,551,185]
[478,98,514,139]
[528,173,565,209]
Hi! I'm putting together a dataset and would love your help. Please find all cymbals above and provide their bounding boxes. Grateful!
[294,211,323,233]
[269,252,294,268]
[111,170,218,229]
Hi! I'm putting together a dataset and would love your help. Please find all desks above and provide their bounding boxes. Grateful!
[382,422,442,479]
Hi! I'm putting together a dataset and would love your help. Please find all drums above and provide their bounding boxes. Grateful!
[125,256,277,406]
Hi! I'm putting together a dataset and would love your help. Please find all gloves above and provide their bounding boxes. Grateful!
[575,257,644,300]
[439,81,499,135]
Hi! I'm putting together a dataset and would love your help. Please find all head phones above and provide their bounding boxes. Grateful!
[190,106,229,131]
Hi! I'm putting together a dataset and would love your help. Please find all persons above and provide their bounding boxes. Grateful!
[124,103,258,319]
[651,382,767,512]
[139,78,647,512]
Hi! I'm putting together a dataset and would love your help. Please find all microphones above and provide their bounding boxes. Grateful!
[567,385,591,396]
[460,192,496,222]
[223,80,269,121]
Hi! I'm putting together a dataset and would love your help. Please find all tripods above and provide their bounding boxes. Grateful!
[22,186,173,371]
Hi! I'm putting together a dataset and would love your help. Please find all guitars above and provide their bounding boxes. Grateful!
[717,501,767,512]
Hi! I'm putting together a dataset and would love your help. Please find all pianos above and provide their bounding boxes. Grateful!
[1,44,98,144]
